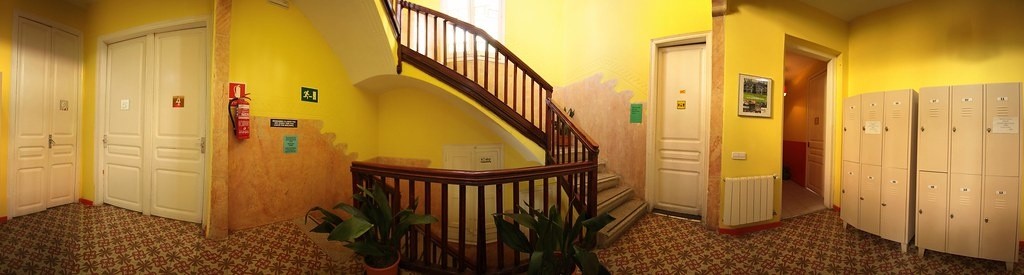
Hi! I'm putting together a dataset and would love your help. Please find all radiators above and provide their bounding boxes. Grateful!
[722,174,779,227]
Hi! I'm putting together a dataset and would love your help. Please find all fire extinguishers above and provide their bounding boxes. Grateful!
[229,93,252,140]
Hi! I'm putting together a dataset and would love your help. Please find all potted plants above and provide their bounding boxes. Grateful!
[491,197,616,275]
[304,172,438,275]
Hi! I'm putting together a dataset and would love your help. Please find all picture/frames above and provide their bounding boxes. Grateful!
[738,74,772,117]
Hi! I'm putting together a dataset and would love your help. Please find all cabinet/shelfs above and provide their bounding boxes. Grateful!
[839,83,1024,269]
